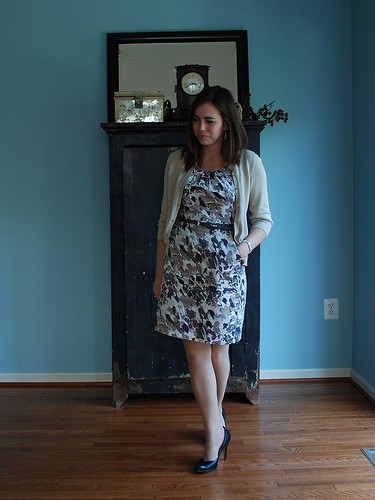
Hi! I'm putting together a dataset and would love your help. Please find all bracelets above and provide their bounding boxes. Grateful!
[242,239,253,253]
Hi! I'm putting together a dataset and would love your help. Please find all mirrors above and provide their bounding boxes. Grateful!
[106,29,251,119]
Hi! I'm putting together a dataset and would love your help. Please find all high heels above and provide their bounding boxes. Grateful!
[192,426,231,474]
[200,407,226,443]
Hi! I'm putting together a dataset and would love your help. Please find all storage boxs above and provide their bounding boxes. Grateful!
[112,90,165,124]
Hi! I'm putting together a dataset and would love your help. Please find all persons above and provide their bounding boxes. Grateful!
[153,84,273,474]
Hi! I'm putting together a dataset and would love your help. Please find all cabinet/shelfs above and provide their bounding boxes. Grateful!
[99,121,267,407]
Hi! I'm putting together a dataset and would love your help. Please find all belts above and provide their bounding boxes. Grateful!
[176,217,234,231]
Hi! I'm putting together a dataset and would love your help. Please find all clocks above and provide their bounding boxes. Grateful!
[173,64,212,110]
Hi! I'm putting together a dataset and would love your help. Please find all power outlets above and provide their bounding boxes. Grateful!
[324,299,339,320]
